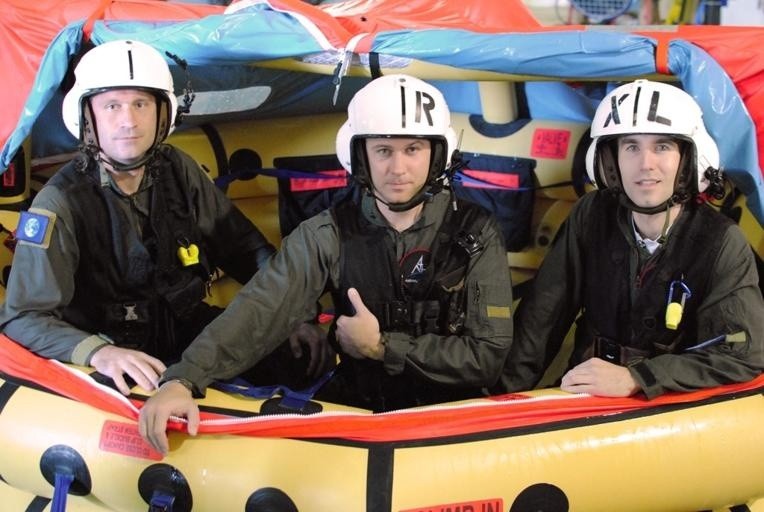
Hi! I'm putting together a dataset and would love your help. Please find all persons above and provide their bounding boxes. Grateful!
[136,74,516,457]
[488,80,764,397]
[0,38,331,396]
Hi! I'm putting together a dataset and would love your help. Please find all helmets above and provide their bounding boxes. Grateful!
[63,39,180,140]
[585,80,721,194]
[335,75,458,175]
